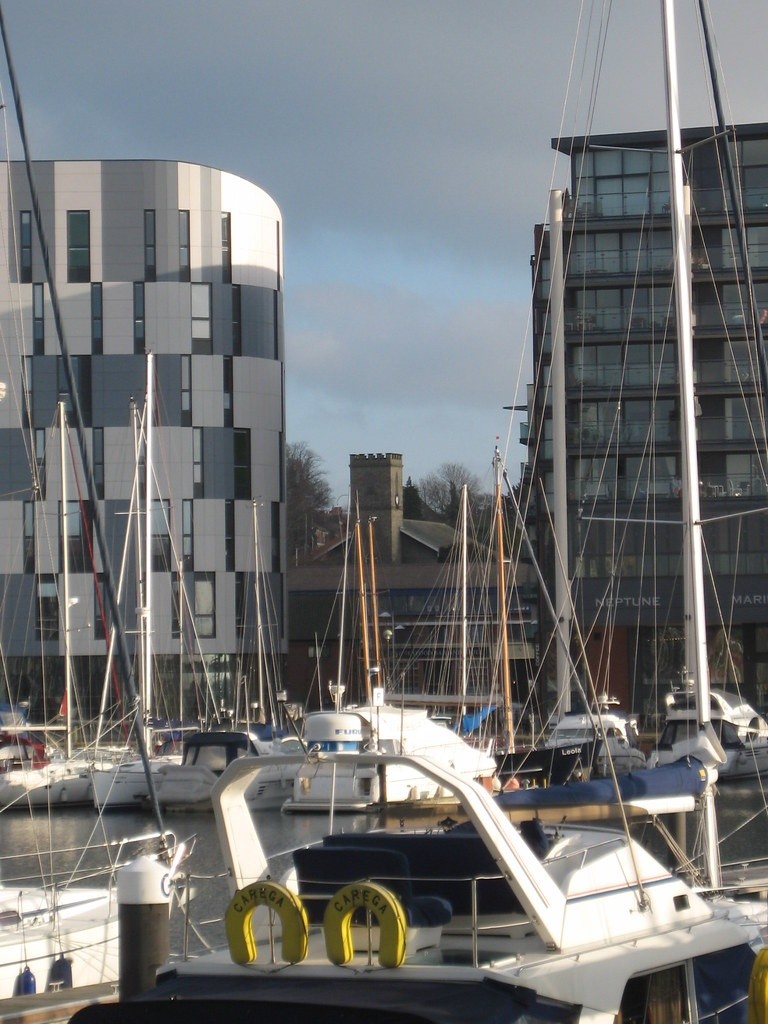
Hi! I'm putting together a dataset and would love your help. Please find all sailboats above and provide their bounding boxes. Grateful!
[0,0,768,1024]
